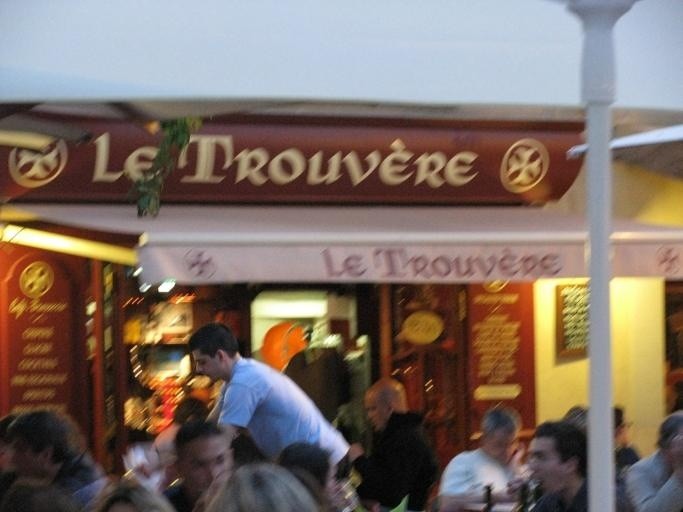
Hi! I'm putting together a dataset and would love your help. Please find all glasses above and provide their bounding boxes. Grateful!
[617,421,633,431]
[174,455,225,471]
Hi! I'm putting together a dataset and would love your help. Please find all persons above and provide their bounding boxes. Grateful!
[349,376,440,512]
[188,322,352,493]
[3,410,110,512]
[0,413,22,512]
[436,407,534,512]
[620,410,683,512]
[528,421,637,512]
[275,442,335,512]
[162,416,235,512]
[610,404,641,471]
[149,396,208,470]
[192,463,318,512]
[91,482,175,512]
[563,404,592,437]
